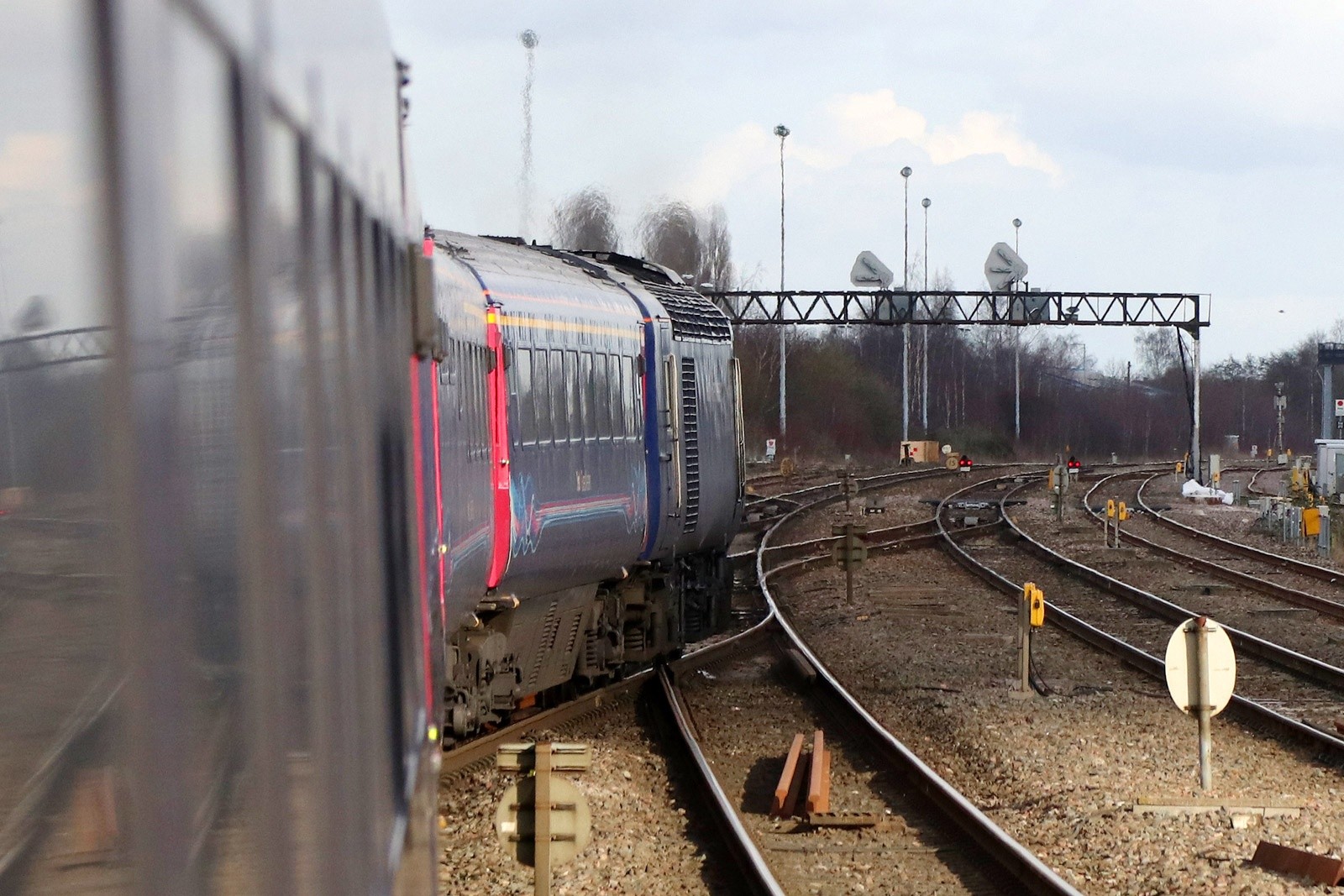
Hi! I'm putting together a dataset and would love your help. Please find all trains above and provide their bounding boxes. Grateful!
[0,1,750,896]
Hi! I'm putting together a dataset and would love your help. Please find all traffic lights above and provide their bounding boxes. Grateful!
[1067,456,1081,468]
[958,455,973,468]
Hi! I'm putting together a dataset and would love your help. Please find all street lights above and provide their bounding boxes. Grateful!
[1013,217,1022,437]
[921,198,933,435]
[772,127,790,446]
[517,26,540,241]
[900,166,913,441]
